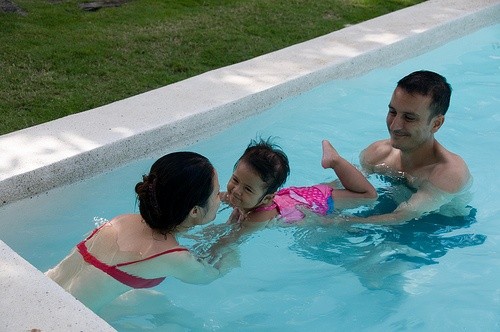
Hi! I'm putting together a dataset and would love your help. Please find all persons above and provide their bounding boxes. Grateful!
[46,151,241,311]
[201,133,378,266]
[277,70,471,226]
